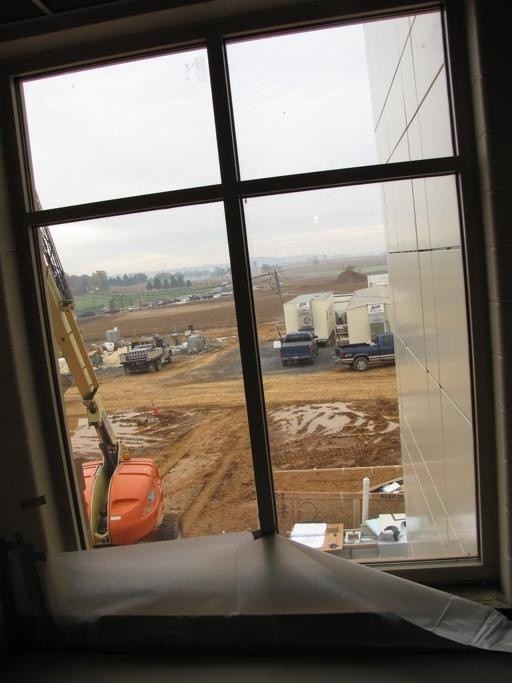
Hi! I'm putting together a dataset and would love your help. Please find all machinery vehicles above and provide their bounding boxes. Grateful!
[32,184,183,546]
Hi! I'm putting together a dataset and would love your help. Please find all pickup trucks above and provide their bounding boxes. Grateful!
[333,330,395,371]
[278,330,320,365]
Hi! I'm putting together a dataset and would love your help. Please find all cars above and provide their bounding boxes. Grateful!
[77,279,230,322]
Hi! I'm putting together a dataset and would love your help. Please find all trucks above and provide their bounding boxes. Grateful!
[120,334,173,374]
